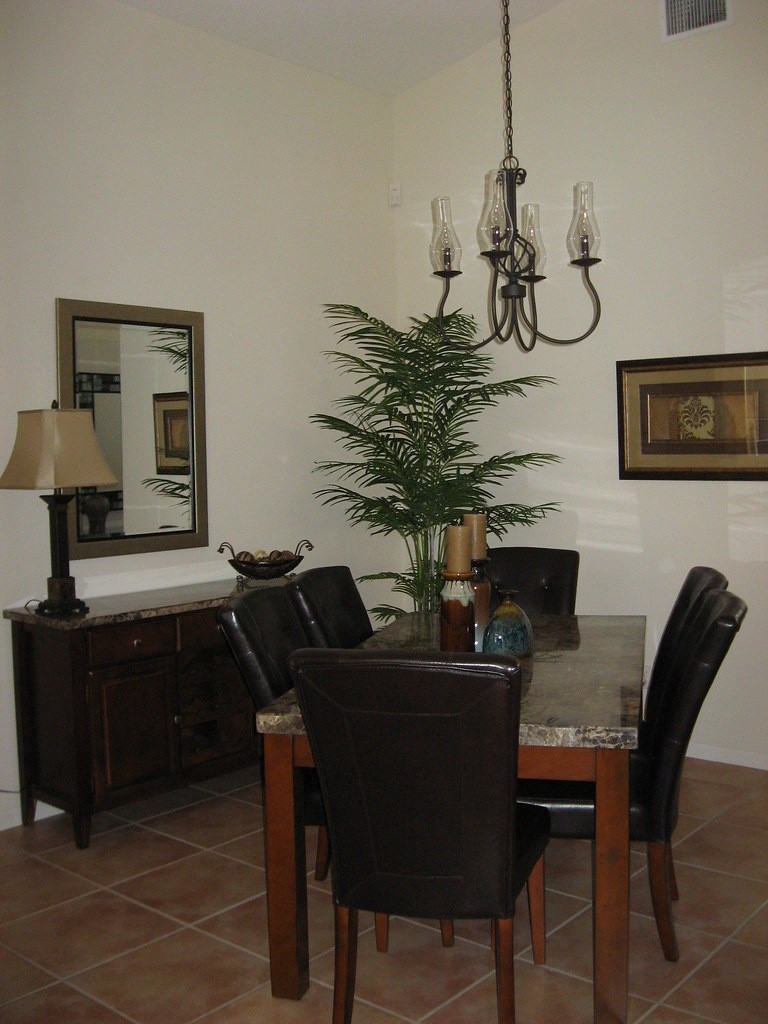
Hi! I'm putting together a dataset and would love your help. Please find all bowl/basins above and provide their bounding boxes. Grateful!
[228,555,304,580]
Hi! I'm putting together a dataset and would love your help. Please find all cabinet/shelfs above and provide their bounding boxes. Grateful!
[2,575,294,851]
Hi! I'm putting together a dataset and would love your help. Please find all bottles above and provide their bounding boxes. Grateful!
[482,590,533,660]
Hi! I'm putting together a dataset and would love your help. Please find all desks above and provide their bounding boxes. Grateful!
[254,611,644,1024]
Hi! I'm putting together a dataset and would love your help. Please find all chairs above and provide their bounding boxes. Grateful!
[628,565,730,765]
[516,591,749,965]
[291,564,374,651]
[290,648,549,1024]
[220,583,330,885]
[467,546,580,616]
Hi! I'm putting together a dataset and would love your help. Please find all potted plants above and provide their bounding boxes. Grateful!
[308,302,565,653]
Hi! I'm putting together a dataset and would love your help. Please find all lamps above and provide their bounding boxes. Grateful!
[430,0,603,353]
[0,400,119,617]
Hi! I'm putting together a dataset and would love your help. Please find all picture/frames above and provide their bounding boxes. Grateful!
[152,391,190,475]
[616,352,768,480]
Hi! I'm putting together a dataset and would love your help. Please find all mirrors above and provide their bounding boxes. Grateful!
[56,297,209,561]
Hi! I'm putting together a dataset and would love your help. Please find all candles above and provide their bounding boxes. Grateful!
[462,513,487,560]
[448,526,471,574]
